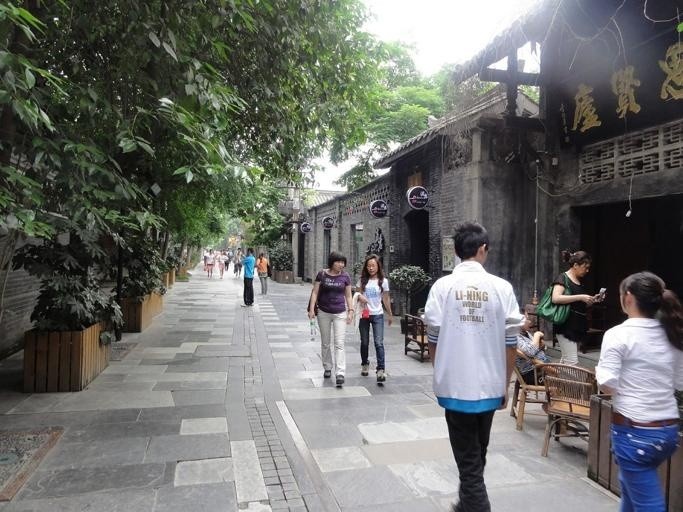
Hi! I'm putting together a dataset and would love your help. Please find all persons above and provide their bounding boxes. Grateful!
[202,248,245,279]
[308,252,354,387]
[355,254,392,386]
[424,220,525,511]
[552,251,605,368]
[513,304,554,385]
[240,248,255,307]
[595,270,682,511]
[256,253,269,294]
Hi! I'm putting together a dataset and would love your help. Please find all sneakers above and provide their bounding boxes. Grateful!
[335,374,344,384]
[323,369,331,377]
[376,370,387,384]
[361,360,369,375]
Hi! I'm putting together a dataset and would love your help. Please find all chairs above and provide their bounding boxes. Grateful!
[533,362,598,456]
[509,347,561,429]
[402,313,431,362]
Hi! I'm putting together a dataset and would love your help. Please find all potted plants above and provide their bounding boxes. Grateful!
[268,240,293,284]
[3,240,126,393]
[151,235,187,321]
[389,265,429,334]
[587,393,683,512]
[113,235,171,333]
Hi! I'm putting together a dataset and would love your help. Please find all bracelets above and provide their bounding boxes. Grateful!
[348,309,354,312]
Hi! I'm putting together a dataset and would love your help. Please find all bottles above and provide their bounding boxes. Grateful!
[310,317,316,336]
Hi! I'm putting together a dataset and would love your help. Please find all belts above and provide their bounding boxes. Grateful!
[611,412,679,429]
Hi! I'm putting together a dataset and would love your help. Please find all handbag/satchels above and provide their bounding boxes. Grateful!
[534,282,571,324]
[307,298,318,317]
